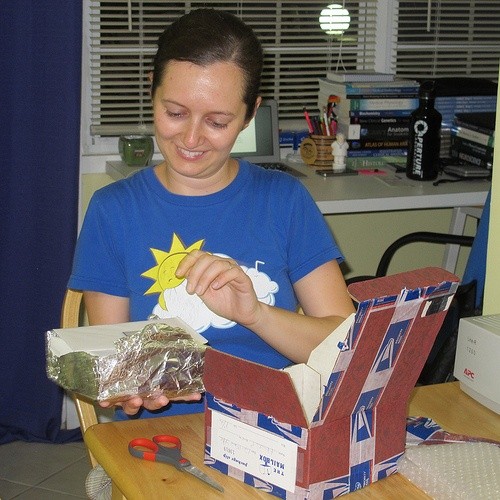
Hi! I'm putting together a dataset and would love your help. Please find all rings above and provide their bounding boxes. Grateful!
[226,260,233,269]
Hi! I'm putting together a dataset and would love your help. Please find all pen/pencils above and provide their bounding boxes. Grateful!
[303,105,336,136]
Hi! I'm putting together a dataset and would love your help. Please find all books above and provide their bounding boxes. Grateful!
[434,96,497,172]
[317,71,419,168]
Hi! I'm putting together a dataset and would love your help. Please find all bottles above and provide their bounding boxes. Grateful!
[405,80,443,182]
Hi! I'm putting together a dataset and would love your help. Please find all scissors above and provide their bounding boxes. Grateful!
[128,434,225,492]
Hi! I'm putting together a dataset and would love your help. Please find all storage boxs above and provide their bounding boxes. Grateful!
[46,316,208,403]
[453,313,500,417]
[204,267,459,499]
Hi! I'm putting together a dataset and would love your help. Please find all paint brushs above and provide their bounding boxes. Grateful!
[326,94,340,117]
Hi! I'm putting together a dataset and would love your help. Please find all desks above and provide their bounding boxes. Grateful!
[83,381,500,500]
[81,159,490,280]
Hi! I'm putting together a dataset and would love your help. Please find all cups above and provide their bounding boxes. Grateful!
[310,134,337,171]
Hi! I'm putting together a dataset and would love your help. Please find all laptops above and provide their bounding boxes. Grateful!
[229,99,308,180]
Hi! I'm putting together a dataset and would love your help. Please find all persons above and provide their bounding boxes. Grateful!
[67,10,356,421]
[330,133,349,169]
